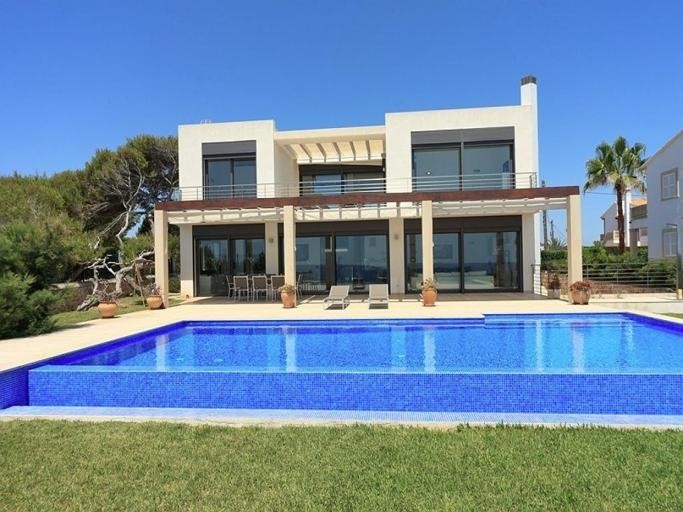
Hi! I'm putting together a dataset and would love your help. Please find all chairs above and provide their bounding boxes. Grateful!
[225,272,305,303]
[322,284,351,309]
[368,283,389,308]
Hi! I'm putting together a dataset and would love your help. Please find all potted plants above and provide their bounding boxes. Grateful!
[145,284,163,308]
[547,276,561,299]
[278,284,296,308]
[417,277,440,306]
[97,293,119,318]
[569,280,594,305]
